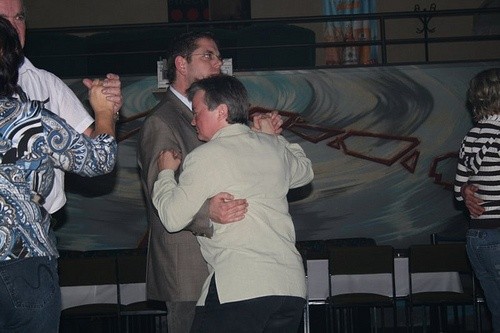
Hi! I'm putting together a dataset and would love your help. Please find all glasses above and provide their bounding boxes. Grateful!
[184,53,223,61]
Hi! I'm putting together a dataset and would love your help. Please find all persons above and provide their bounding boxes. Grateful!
[451,68,500,331]
[0,0,122,214]
[137,30,283,333]
[0,16,117,333]
[151,74,315,332]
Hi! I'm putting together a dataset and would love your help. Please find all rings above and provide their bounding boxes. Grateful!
[261,115,265,118]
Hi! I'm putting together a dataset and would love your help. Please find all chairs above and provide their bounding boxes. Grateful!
[56,249,168,333]
[295,237,488,333]
